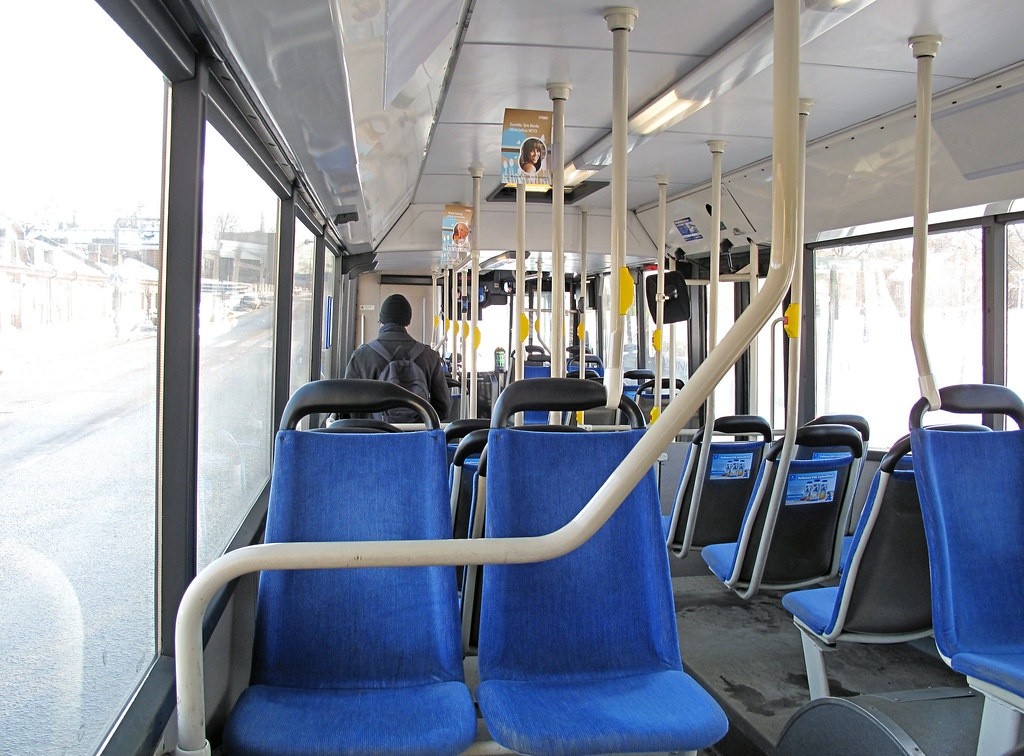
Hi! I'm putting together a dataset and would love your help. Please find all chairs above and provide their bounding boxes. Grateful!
[222,347,1024,756]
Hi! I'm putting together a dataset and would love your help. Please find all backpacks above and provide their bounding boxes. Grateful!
[365,338,431,423]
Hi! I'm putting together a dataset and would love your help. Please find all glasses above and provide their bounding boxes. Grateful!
[377,319,382,324]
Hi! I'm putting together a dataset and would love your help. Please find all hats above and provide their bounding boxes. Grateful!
[379,294,413,327]
[450,362,463,368]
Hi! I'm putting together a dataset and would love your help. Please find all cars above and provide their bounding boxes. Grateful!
[239,294,262,310]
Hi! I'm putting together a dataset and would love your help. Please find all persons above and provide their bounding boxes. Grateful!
[521,139,542,173]
[345,294,453,424]
[458,224,469,244]
